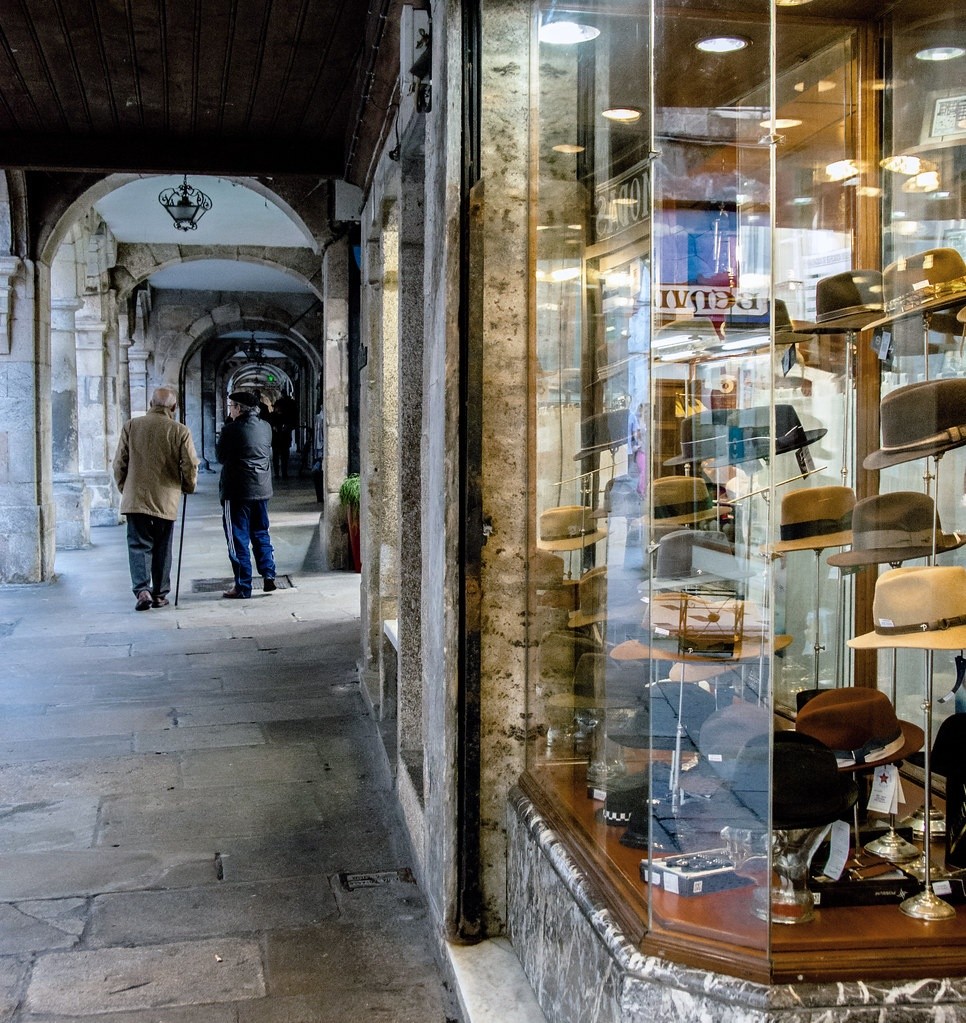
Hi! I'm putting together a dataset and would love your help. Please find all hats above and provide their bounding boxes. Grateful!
[228,392,258,407]
[860,246,966,333]
[793,268,916,334]
[572,408,647,462]
[663,409,737,466]
[826,490,966,567]
[535,505,608,551]
[533,529,966,851]
[759,486,856,553]
[573,410,646,462]
[630,476,732,526]
[583,335,662,392]
[702,404,827,468]
[588,474,649,519]
[863,377,966,471]
[590,272,642,321]
[703,297,816,354]
[628,317,757,362]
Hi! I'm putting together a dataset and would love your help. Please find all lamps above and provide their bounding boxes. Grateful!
[246,340,264,362]
[158,175,213,230]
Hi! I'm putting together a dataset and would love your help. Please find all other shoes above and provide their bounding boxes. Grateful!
[263,576,276,592]
[135,590,153,611]
[152,598,169,608]
[223,589,251,598]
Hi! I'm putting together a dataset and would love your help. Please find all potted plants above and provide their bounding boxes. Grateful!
[338,472,362,572]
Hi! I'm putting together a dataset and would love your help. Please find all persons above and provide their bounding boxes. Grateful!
[252,389,297,477]
[214,392,277,598]
[111,388,198,611]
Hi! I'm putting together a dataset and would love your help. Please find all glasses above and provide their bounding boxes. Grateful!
[226,402,243,410]
